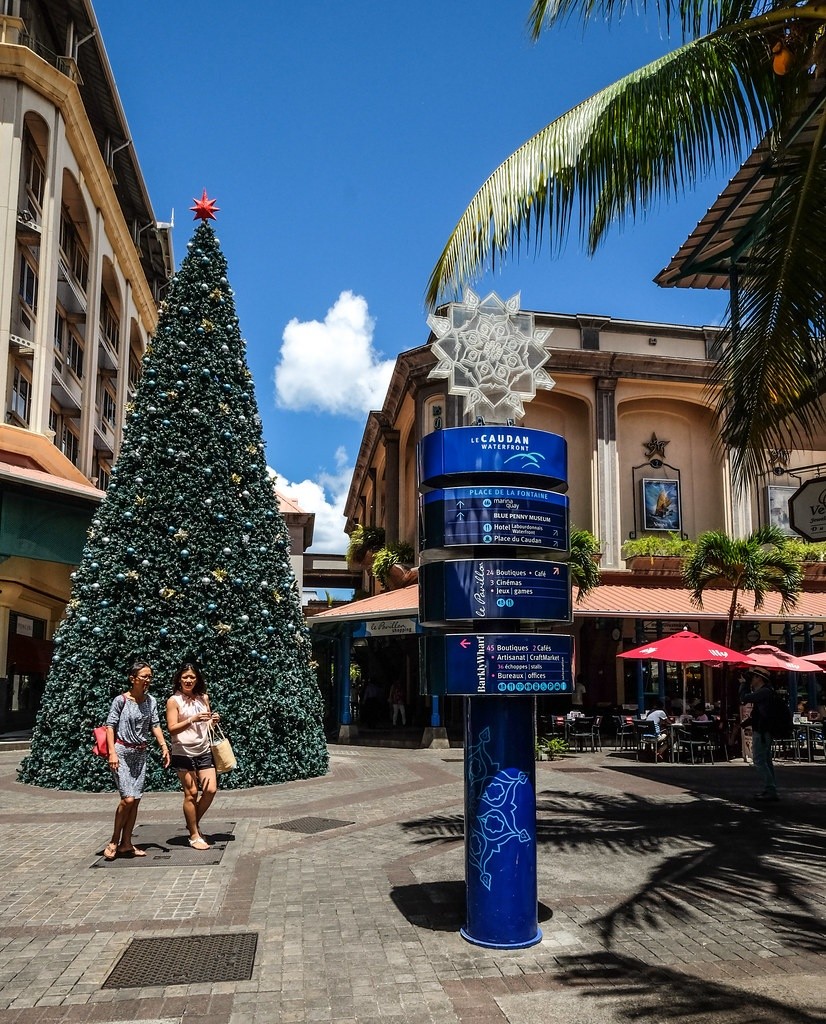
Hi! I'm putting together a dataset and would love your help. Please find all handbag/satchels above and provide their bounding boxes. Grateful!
[207,718,239,774]
[92,725,116,758]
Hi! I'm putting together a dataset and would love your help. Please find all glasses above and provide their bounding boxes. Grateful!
[136,674,154,683]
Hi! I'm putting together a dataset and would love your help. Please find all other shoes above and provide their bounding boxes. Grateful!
[753,787,778,800]
[656,753,664,762]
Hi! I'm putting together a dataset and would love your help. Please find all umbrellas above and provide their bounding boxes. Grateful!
[799,652,826,664]
[616,626,756,714]
[701,641,826,672]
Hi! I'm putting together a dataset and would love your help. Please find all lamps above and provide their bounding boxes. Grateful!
[17,208,33,222]
[43,429,57,438]
[89,475,99,486]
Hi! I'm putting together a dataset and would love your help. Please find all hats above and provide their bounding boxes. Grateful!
[748,666,771,682]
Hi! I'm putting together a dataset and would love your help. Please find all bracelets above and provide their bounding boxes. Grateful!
[163,749,166,751]
[161,743,166,747]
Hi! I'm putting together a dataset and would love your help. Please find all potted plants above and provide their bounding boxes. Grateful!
[371,542,415,590]
[622,532,826,574]
[344,520,385,574]
[583,529,603,564]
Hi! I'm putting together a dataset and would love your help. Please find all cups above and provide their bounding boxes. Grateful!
[793,716,807,724]
[567,711,585,719]
[670,717,683,723]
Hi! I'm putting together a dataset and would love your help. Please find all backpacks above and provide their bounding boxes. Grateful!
[762,685,794,739]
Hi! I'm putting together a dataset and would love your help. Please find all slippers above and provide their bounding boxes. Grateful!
[104,843,118,861]
[115,846,146,857]
[185,824,210,851]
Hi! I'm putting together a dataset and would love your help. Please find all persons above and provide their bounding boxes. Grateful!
[103,664,170,861]
[390,677,408,728]
[695,704,708,732]
[643,701,672,760]
[572,675,586,710]
[736,667,780,801]
[350,681,384,717]
[166,663,220,850]
[740,703,754,762]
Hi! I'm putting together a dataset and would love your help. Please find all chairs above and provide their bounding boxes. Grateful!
[551,710,826,764]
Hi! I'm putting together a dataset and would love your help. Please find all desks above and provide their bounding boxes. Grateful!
[666,723,691,762]
[793,724,824,763]
[554,719,580,746]
